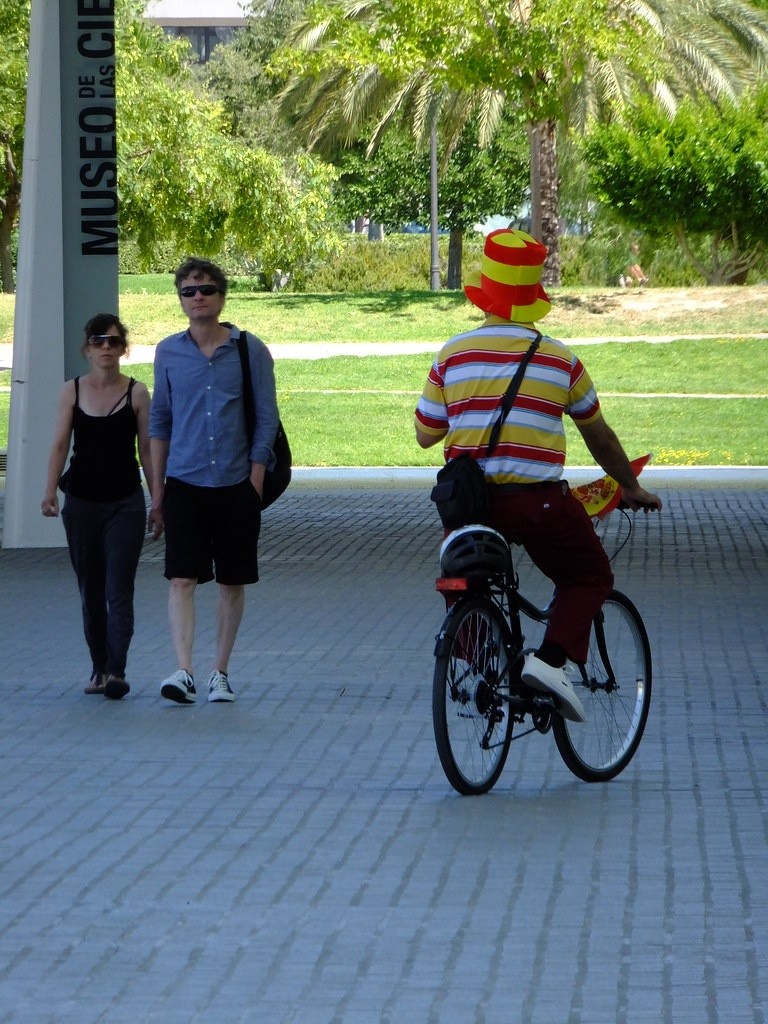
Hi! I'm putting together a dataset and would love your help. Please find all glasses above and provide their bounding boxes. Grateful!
[87,334,123,348]
[180,285,222,297]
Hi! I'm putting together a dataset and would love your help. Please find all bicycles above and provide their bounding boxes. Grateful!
[431,494,661,795]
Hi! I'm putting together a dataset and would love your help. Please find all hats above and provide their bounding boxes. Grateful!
[462,229,552,324]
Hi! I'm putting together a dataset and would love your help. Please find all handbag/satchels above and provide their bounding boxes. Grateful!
[238,331,292,510]
[429,452,490,529]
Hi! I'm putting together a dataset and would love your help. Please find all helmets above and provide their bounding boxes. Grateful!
[438,524,512,580]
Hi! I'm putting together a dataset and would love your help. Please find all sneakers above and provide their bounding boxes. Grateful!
[521,652,586,723]
[205,670,235,702]
[160,669,196,704]
[104,674,130,699]
[84,669,106,694]
[451,657,505,699]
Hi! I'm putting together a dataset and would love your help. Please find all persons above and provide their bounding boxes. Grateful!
[415,230,662,725]
[41,314,152,700]
[146,257,278,703]
